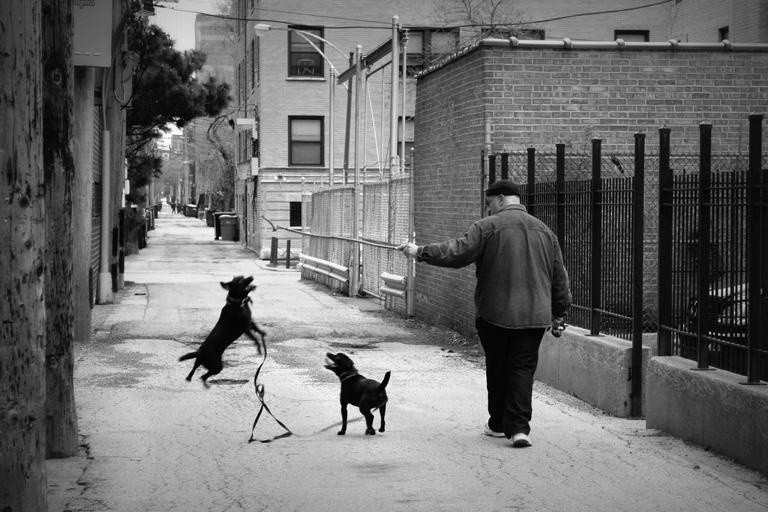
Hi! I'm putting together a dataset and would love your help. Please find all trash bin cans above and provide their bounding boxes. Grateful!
[146,210,154,229]
[205,208,215,226]
[153,205,162,218]
[186,204,197,216]
[214,211,238,242]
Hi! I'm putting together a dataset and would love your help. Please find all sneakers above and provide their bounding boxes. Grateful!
[483,422,532,447]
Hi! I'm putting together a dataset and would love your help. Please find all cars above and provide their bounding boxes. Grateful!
[678,282,767,376]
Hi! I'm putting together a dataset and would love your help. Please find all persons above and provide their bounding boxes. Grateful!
[395,177,572,446]
[176,204,181,215]
[170,200,176,215]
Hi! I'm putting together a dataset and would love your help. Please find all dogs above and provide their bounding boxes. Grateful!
[179,276,267,384]
[324,352,391,435]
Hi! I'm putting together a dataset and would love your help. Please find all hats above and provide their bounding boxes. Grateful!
[485,181,521,197]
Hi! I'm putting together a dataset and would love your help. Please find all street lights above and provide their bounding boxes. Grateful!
[254,23,354,183]
